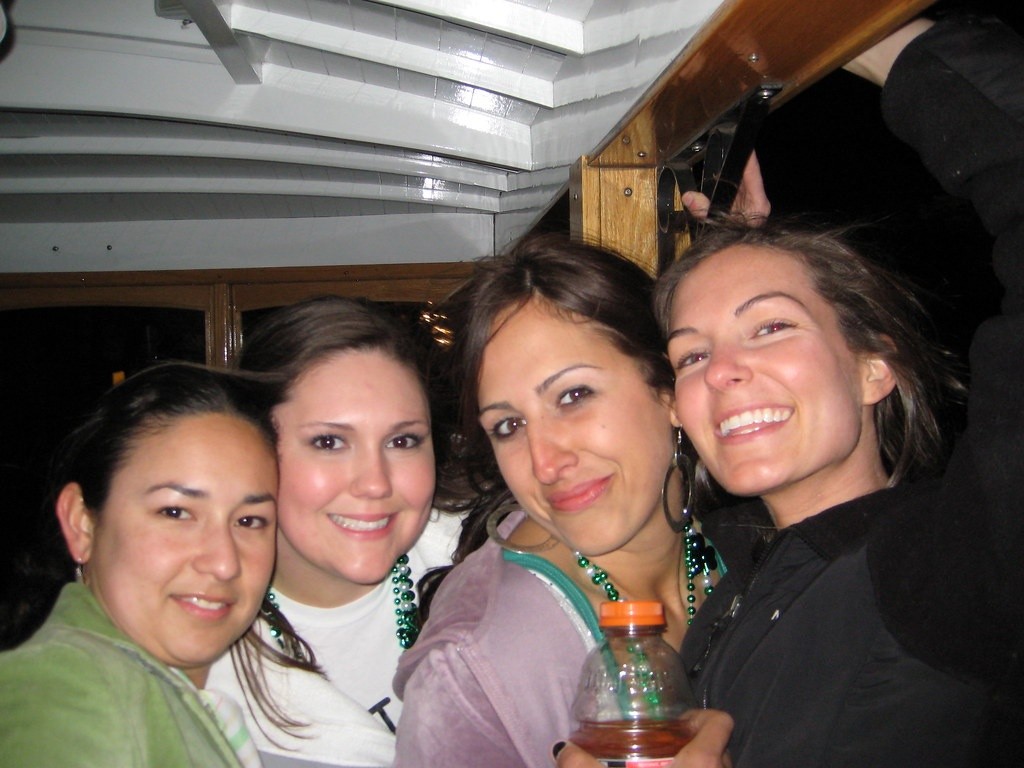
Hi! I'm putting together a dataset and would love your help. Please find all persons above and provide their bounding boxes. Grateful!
[204,294,514,768]
[678,2,1022,525]
[389,234,725,768]
[0,359,277,768]
[652,222,984,768]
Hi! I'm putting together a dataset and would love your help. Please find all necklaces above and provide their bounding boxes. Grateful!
[259,555,416,701]
[561,521,717,717]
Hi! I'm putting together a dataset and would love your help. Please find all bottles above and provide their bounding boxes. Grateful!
[568,600,697,768]
